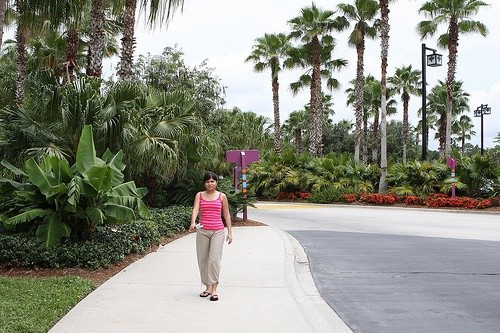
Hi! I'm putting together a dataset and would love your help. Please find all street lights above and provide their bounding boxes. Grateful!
[422,44,442,163]
[475,104,491,155]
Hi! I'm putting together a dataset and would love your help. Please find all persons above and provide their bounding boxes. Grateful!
[190,173,232,301]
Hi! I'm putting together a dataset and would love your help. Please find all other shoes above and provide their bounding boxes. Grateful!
[210,294,218,300]
[200,290,211,296]
